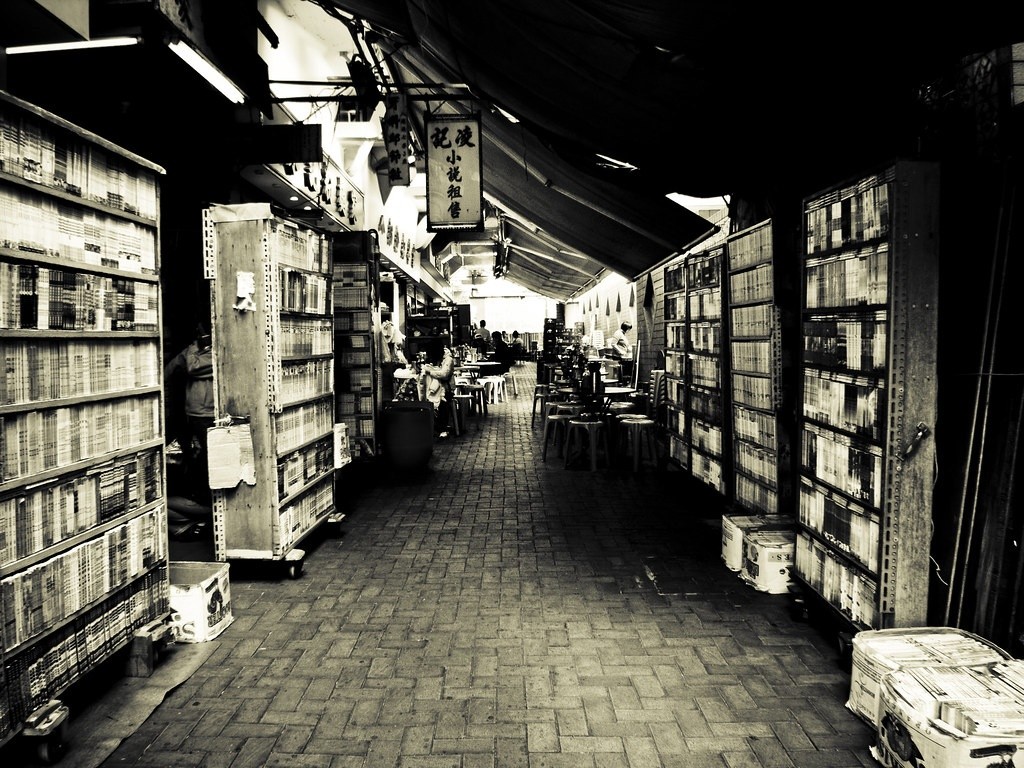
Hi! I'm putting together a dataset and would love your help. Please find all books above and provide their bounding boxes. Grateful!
[0,117,171,744]
[663,182,891,629]
[269,216,374,554]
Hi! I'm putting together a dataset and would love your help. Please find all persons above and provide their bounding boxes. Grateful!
[501,331,510,343]
[480,330,511,378]
[165,312,214,506]
[476,318,491,341]
[611,321,633,358]
[419,336,454,438]
[509,332,524,366]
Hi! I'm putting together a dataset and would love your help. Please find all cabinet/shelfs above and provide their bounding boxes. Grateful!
[0,87,1024,766]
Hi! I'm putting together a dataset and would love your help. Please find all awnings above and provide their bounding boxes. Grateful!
[382,24,723,305]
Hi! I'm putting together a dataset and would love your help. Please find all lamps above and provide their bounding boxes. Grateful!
[0,36,144,55]
[165,36,246,108]
